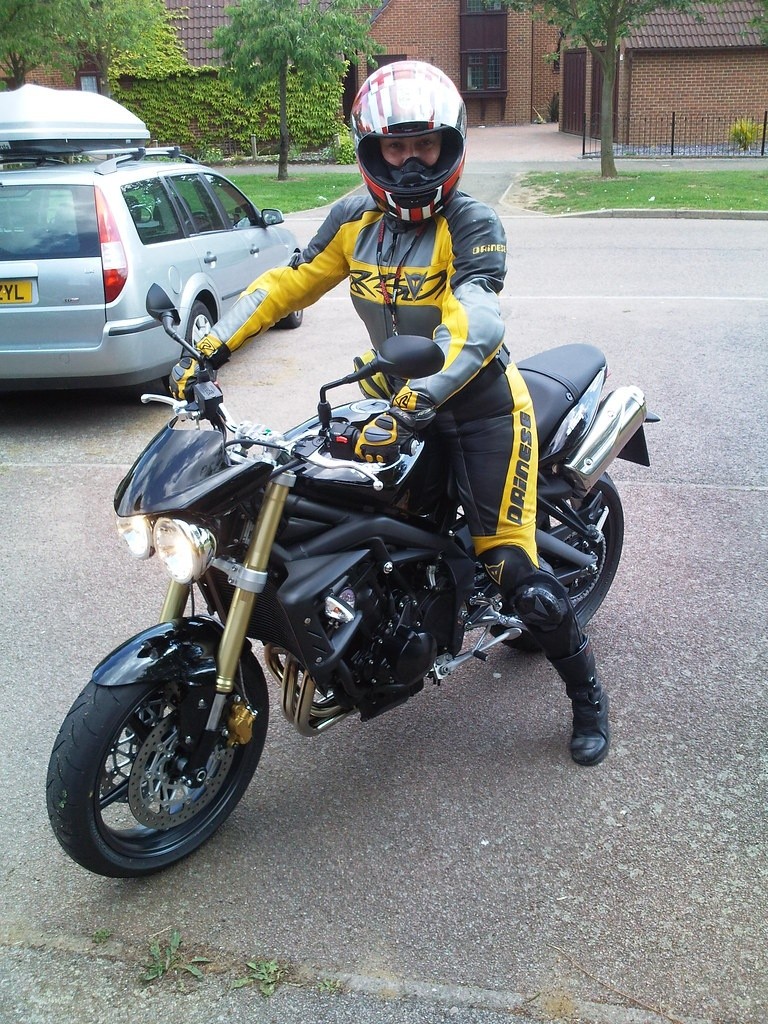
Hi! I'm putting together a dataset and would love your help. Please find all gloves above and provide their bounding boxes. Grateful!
[354,387,436,464]
[168,334,231,403]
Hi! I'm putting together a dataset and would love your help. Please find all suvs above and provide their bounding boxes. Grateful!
[1,85,308,401]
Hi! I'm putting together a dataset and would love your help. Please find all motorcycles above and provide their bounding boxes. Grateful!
[44,280,663,879]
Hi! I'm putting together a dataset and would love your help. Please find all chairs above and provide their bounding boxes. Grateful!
[152,196,193,231]
[124,195,141,221]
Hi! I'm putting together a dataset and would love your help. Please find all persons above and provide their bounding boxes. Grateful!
[161,58,614,770]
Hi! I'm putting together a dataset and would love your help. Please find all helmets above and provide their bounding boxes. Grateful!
[350,61,468,223]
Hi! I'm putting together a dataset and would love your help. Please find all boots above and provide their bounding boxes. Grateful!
[547,632,611,767]
